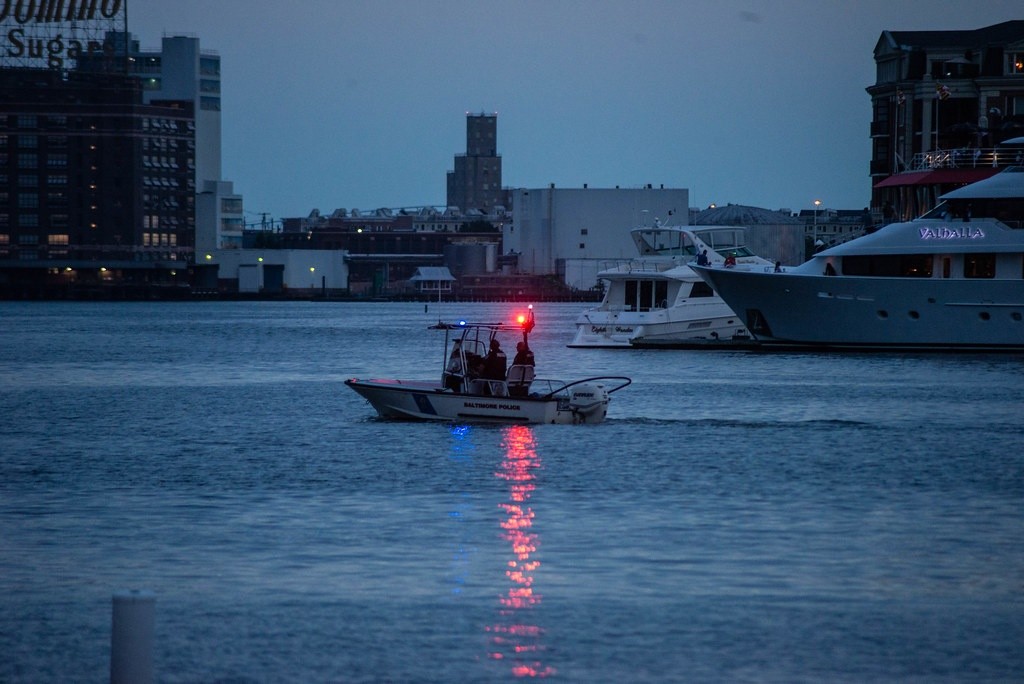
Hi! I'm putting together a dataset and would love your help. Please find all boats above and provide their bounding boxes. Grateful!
[568,217,833,350]
[344,317,632,424]
[687,138,1023,352]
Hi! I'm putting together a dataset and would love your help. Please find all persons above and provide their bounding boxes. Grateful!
[479,339,507,381]
[507,342,536,396]
[775,261,782,272]
[723,253,736,268]
[823,263,836,276]
[697,249,711,266]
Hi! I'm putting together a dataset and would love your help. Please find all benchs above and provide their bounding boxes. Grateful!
[507,365,535,395]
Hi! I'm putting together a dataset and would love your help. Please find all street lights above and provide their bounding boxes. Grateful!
[813,200,821,248]
